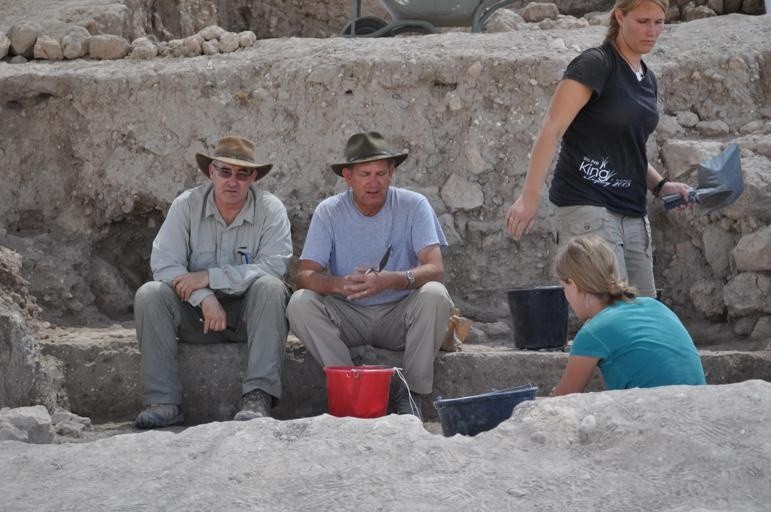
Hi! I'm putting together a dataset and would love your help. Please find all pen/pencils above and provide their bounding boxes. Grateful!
[242,252,248,264]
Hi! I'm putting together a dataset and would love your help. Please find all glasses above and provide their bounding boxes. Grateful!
[212,162,252,181]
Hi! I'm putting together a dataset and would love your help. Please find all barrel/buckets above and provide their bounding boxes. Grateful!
[504,285,568,351]
[433,383,539,438]
[323,364,393,419]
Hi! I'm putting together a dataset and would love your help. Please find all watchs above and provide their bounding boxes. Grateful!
[404,270,416,290]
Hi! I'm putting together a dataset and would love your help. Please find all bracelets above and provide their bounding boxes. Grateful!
[652,177,668,196]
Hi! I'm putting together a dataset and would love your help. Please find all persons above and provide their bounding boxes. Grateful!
[549,234,705,395]
[506,0,693,301]
[287,129,453,424]
[134,136,294,429]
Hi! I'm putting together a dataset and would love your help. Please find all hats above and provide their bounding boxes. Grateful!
[195,136,273,181]
[331,132,409,177]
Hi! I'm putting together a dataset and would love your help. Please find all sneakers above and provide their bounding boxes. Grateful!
[234,389,273,421]
[135,402,184,428]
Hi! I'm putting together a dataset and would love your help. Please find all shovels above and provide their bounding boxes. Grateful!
[663,144,745,218]
[365,245,392,275]
